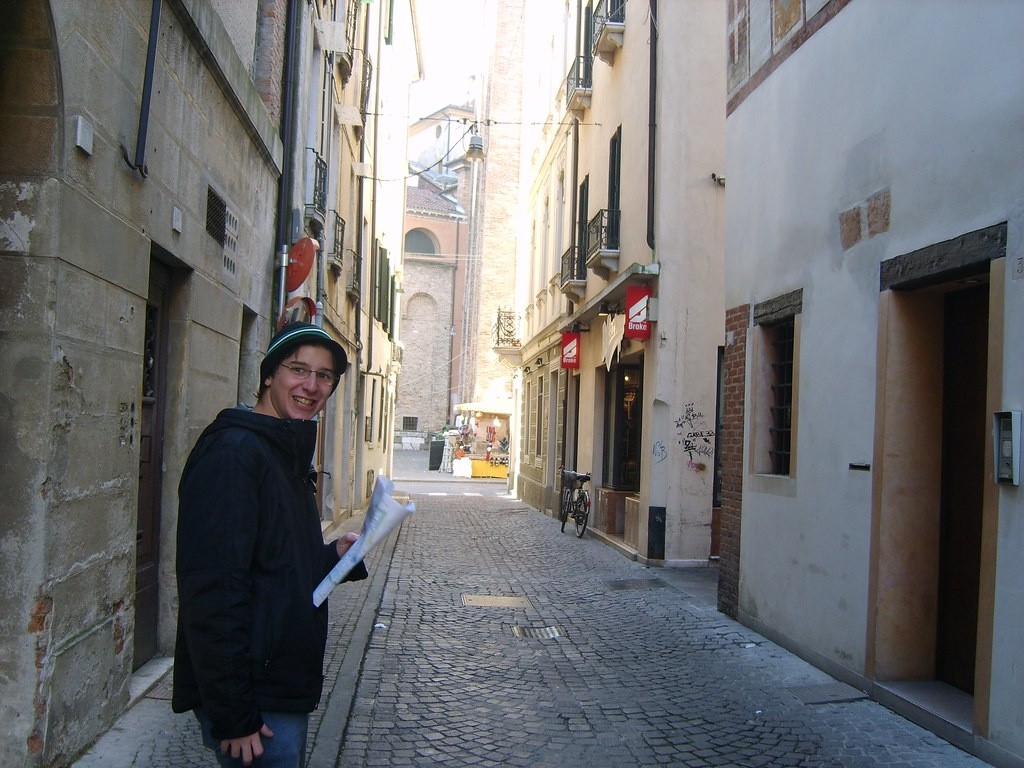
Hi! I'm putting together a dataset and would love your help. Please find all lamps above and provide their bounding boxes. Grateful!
[597,304,620,317]
[534,358,542,364]
[523,367,530,372]
[465,122,485,164]
[570,323,590,333]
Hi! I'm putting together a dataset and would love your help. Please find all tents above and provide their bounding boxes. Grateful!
[453,398,513,428]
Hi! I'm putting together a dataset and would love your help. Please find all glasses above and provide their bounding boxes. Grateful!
[277,363,339,386]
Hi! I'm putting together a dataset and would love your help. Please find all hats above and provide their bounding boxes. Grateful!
[259,320,348,397]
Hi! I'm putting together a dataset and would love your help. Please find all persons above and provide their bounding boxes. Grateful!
[171,323,369,768]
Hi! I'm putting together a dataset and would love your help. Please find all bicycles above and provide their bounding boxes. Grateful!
[557,464,592,538]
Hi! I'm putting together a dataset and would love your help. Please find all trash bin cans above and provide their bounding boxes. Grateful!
[428,440,445,470]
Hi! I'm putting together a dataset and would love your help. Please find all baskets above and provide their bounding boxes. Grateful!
[562,470,582,489]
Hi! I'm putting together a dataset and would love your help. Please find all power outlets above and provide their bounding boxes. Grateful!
[173,205,182,233]
[75,114,94,157]
